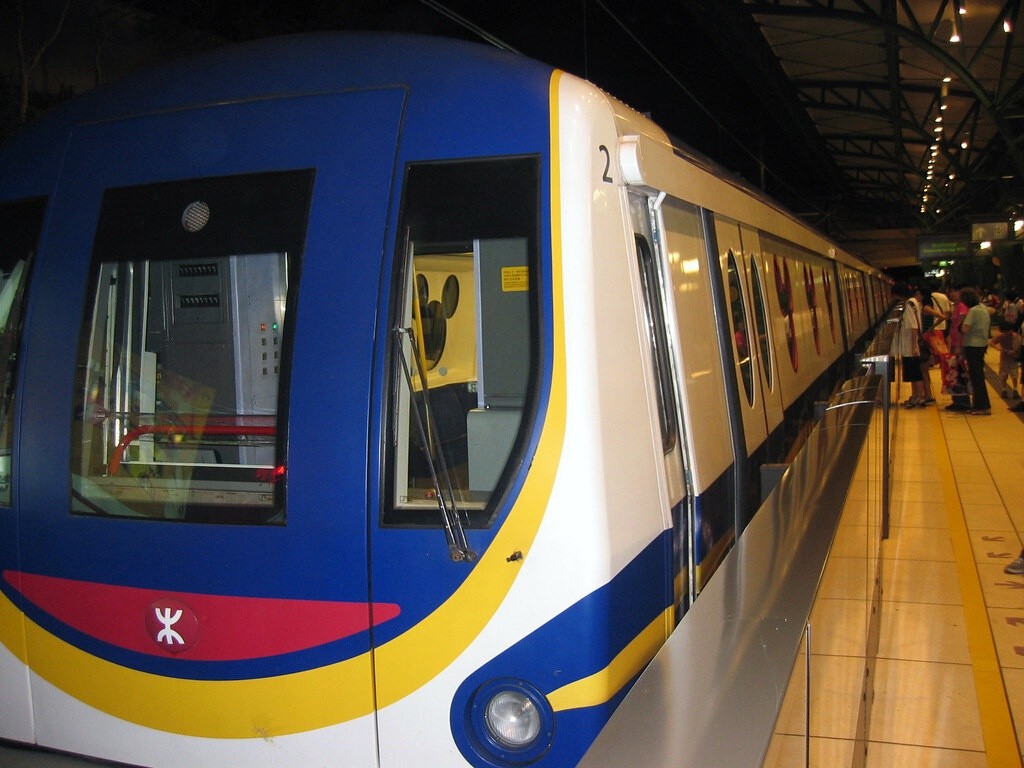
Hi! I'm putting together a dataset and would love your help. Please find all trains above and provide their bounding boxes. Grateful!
[0,32,901,767]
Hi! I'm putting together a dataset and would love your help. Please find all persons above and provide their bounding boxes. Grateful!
[890,281,1024,416]
[733,293,834,362]
[1004,549,1024,574]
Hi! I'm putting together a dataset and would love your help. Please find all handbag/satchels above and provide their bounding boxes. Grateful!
[918,336,931,363]
[940,353,972,396]
[924,329,948,356]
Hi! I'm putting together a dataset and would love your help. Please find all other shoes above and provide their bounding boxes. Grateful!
[1002,390,1007,398]
[905,396,926,409]
[946,403,969,411]
[967,407,991,414]
[925,399,936,405]
[1013,391,1019,399]
[1008,402,1024,411]
